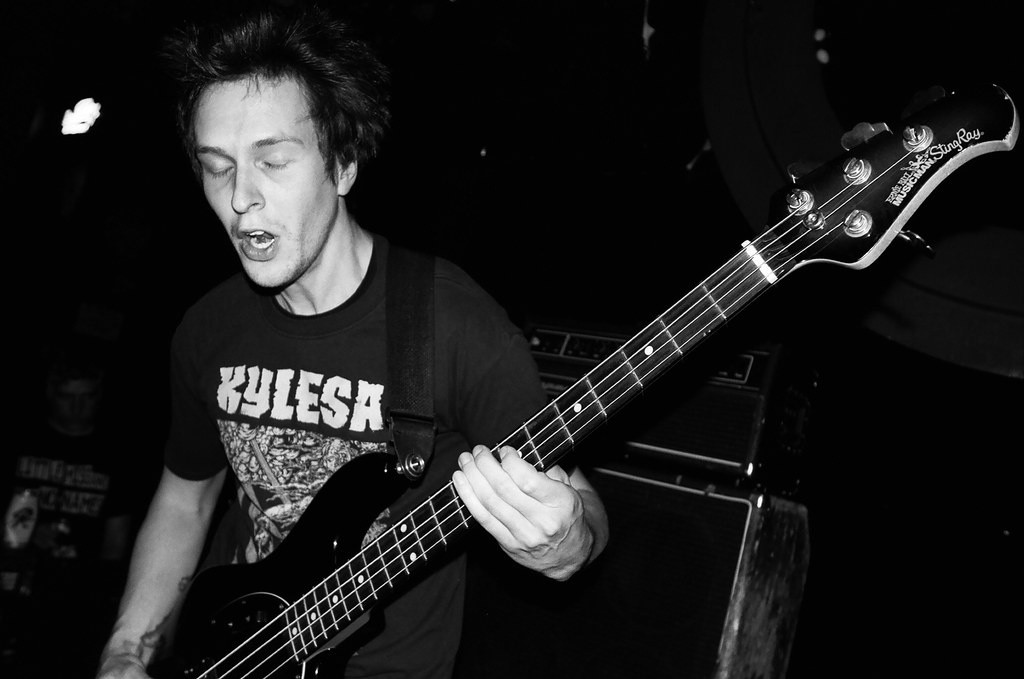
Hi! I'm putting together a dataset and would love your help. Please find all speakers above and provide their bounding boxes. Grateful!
[451,457,811,679]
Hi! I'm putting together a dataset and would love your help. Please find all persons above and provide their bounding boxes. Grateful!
[96,0,610,678]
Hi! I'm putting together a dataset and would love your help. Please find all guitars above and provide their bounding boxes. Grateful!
[151,80,1022,678]
